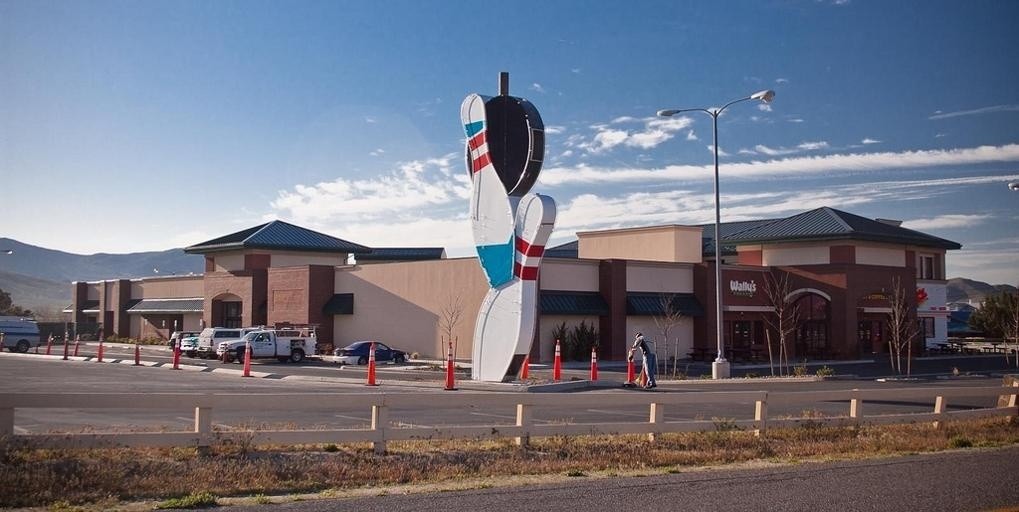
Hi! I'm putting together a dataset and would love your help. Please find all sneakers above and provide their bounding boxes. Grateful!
[646,384,656,388]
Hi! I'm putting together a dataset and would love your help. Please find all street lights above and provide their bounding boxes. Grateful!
[0,249,15,255]
[1006,182,1019,193]
[654,87,778,380]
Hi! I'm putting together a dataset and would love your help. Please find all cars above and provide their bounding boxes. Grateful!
[46,331,65,344]
[330,339,410,367]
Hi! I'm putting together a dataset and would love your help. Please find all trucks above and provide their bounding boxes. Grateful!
[168,325,318,364]
[0,315,43,352]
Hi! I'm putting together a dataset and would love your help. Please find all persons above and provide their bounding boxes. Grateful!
[630,333,657,389]
